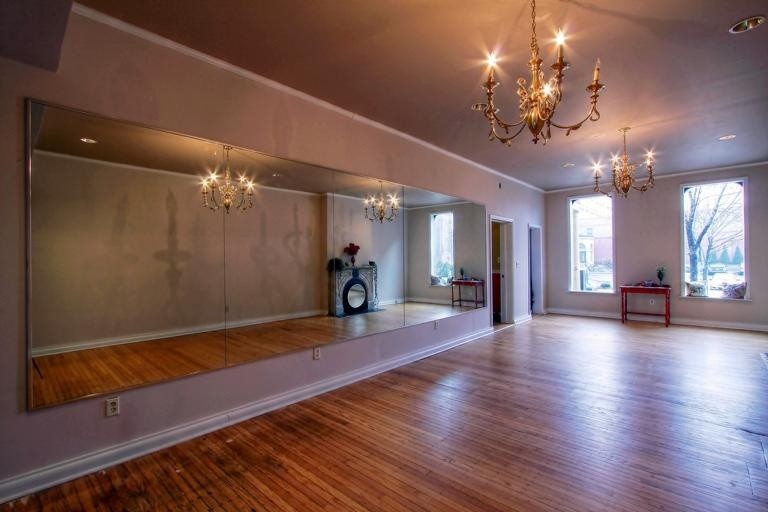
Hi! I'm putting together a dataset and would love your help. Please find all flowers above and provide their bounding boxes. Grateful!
[344,242,361,255]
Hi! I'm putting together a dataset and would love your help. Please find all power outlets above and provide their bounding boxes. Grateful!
[105,397,120,417]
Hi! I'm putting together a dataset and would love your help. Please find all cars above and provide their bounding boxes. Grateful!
[685,262,743,294]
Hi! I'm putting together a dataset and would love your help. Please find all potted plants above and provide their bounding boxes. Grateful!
[656,263,667,286]
[460,267,464,278]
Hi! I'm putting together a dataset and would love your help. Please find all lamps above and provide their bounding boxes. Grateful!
[363,180,402,222]
[592,126,661,198]
[469,1,607,150]
[197,144,258,215]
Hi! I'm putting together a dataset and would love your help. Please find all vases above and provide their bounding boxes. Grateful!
[351,255,356,266]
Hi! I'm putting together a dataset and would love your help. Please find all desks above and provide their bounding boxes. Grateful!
[451,278,485,308]
[619,284,672,326]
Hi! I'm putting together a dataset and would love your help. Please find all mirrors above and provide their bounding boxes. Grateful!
[16,96,492,416]
